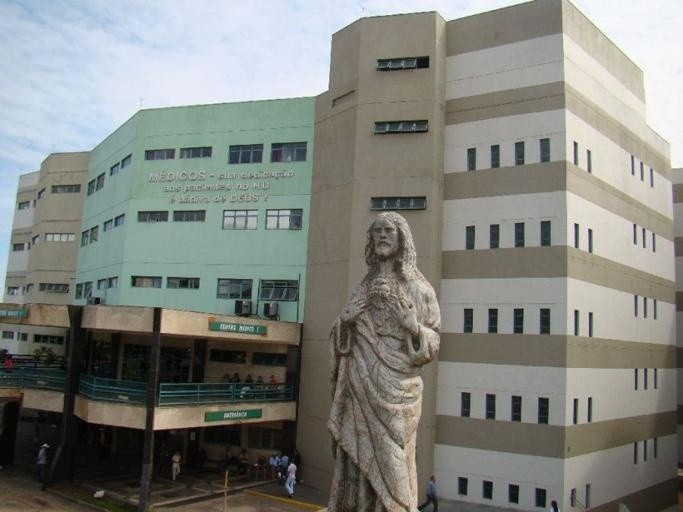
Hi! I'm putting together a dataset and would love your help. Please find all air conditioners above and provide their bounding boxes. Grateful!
[85,296,101,306]
[262,300,279,317]
[233,298,252,316]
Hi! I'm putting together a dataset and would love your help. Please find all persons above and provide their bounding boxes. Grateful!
[3,355,13,385]
[32,430,40,464]
[551,500,560,512]
[327,211,441,512]
[160,441,206,482]
[417,475,438,512]
[222,371,279,400]
[36,441,51,477]
[219,442,302,498]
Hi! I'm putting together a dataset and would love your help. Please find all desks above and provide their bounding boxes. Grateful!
[220,454,288,483]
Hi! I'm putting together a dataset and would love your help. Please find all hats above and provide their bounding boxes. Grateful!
[40,444,49,448]
